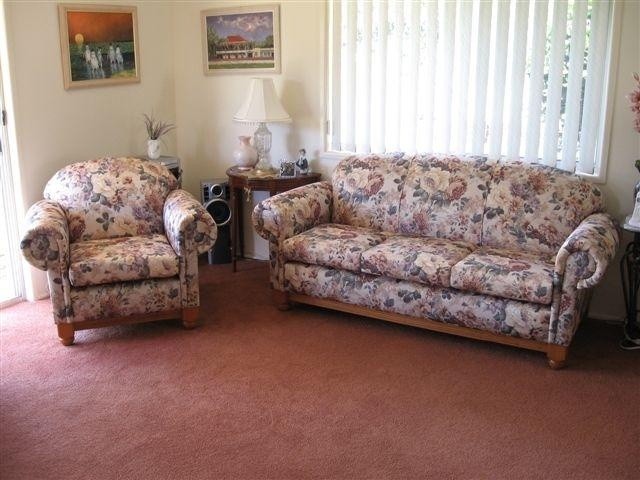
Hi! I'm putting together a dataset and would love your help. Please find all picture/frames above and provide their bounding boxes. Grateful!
[198,3,282,77]
[57,1,141,90]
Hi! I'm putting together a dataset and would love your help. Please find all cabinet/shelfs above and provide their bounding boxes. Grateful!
[618,228,639,351]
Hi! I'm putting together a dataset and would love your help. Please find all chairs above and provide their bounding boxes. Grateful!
[17,154,218,346]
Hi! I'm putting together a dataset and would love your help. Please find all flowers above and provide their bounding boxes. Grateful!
[625,70,639,190]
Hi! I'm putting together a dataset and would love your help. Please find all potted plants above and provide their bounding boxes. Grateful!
[140,109,176,159]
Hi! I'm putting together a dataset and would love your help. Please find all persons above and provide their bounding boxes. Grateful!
[83,41,124,69]
[295,149,308,174]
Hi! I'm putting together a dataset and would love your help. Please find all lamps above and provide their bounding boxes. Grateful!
[233,77,294,175]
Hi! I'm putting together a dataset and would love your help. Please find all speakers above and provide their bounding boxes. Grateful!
[200,177,232,264]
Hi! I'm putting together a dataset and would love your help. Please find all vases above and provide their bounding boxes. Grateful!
[234,134,259,170]
[624,190,640,231]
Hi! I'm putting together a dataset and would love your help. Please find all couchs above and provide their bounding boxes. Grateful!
[251,154,619,368]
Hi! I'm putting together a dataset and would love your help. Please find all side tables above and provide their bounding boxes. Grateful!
[138,155,180,182]
[225,164,323,272]
